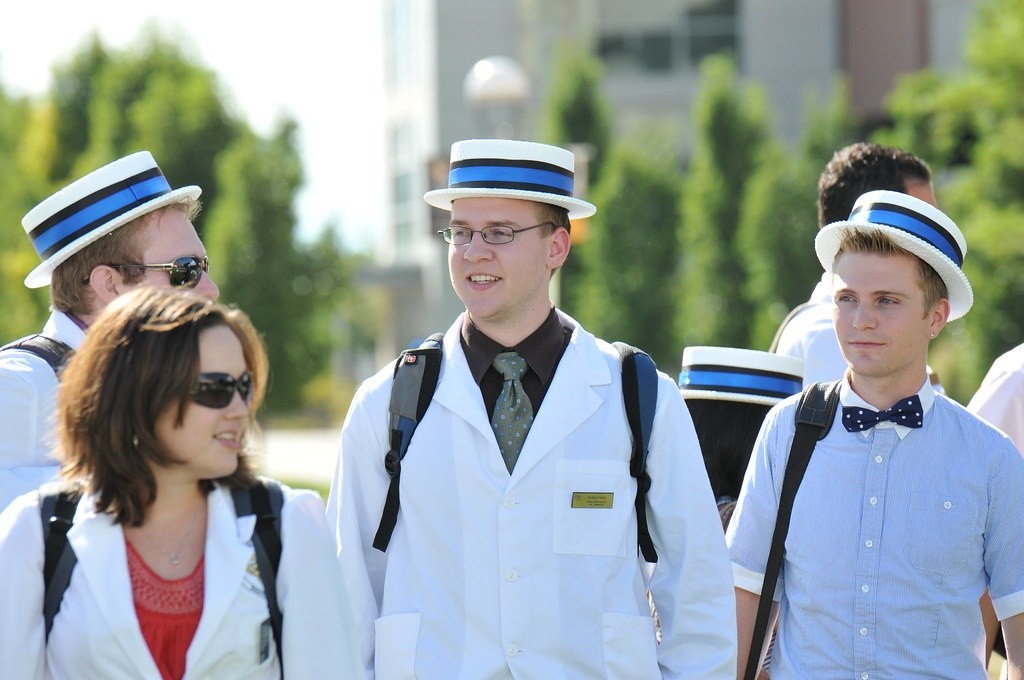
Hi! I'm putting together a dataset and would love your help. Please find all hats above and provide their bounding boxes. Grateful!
[812,191,975,323]
[677,345,807,408]
[18,150,203,289]
[423,139,597,221]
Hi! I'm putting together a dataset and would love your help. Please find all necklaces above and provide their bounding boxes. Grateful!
[141,495,201,565]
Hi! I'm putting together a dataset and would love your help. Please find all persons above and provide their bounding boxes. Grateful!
[0,150,221,513]
[324,138,739,680]
[726,189,1024,677]
[963,340,1024,662]
[0,283,379,680]
[768,140,952,399]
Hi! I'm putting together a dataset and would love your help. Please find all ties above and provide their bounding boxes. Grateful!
[489,351,533,474]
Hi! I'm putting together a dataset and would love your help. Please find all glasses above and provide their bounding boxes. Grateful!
[434,222,558,248]
[194,372,252,408]
[82,257,212,294]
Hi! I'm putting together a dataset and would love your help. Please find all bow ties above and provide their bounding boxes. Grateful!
[841,395,926,434]
